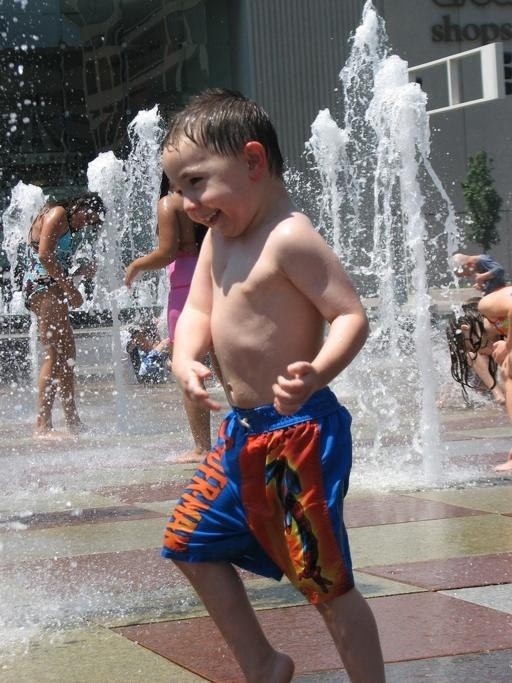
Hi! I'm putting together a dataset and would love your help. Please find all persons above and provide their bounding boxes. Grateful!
[445,286,512,472]
[451,252,506,295]
[24,190,105,441]
[125,170,213,465]
[127,324,170,386]
[159,86,385,683]
[470,354,505,404]
